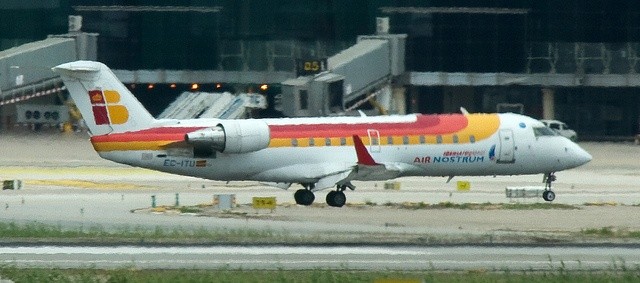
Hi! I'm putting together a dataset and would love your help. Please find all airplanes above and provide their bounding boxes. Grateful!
[51,60,593,208]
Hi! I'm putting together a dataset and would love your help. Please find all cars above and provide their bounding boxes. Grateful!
[541,119,577,142]
